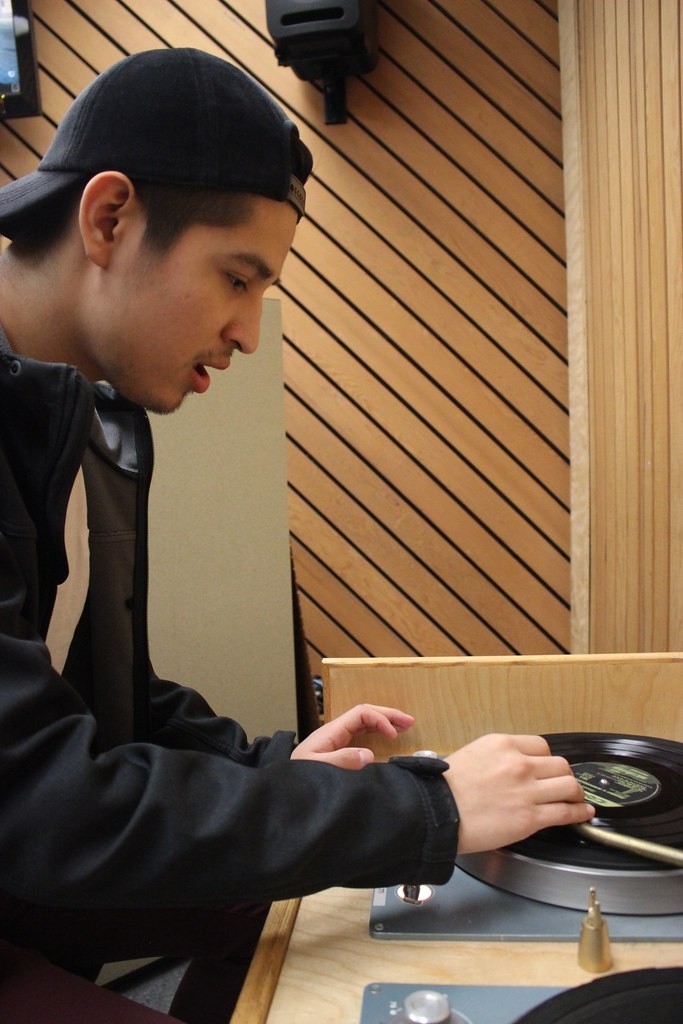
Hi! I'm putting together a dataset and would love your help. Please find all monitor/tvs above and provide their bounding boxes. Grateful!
[1,3,43,122]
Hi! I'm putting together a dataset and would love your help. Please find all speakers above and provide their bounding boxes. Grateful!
[264,0,383,79]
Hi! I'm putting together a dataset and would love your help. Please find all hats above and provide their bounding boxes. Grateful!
[0,47,314,241]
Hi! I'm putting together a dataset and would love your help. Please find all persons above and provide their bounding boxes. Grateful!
[0,47,595,1024]
